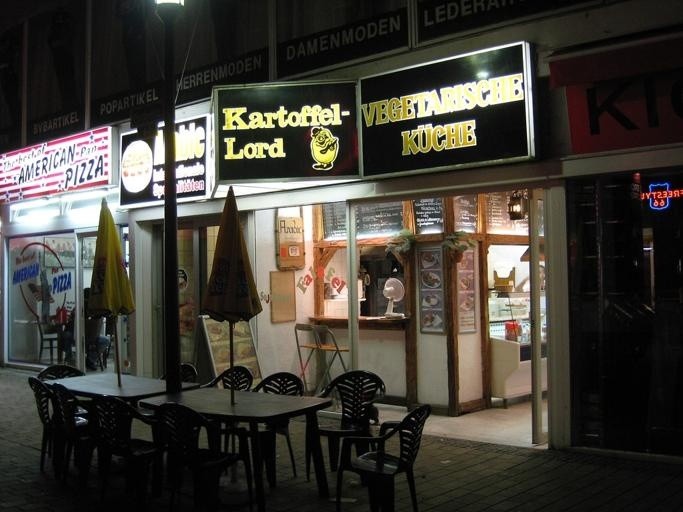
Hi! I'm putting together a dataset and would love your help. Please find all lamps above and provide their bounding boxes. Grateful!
[294,324,348,409]
[84,314,104,371]
[35,322,57,362]
[508,190,528,220]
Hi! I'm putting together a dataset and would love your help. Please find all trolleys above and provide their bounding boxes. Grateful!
[383,277,405,319]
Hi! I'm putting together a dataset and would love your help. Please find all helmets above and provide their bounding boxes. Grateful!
[421,272,441,289]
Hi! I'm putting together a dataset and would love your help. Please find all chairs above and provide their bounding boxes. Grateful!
[304,370,386,484]
[294,324,348,409]
[84,314,104,371]
[152,366,200,449]
[197,366,254,476]
[154,402,254,511]
[35,322,57,362]
[27,363,100,485]
[336,403,430,510]
[232,372,305,479]
[89,395,157,510]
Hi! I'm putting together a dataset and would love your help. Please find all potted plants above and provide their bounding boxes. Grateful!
[43,237,97,269]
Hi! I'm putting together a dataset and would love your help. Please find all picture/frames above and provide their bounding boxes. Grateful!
[43,237,97,269]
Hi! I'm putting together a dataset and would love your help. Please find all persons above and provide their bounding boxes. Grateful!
[59,286,112,372]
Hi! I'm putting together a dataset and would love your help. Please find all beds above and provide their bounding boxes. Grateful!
[198,186,259,406]
[87,197,137,388]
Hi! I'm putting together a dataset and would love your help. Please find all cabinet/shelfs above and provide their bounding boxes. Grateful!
[487,288,547,409]
[56,306,67,326]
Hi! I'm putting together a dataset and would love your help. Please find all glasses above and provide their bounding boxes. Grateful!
[508,190,528,220]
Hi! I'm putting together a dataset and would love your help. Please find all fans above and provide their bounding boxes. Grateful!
[383,277,405,319]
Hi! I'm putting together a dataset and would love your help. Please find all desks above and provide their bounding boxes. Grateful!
[152,0,186,395]
[43,373,200,458]
[140,387,332,510]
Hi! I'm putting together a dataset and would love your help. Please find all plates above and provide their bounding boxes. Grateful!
[487,288,547,409]
